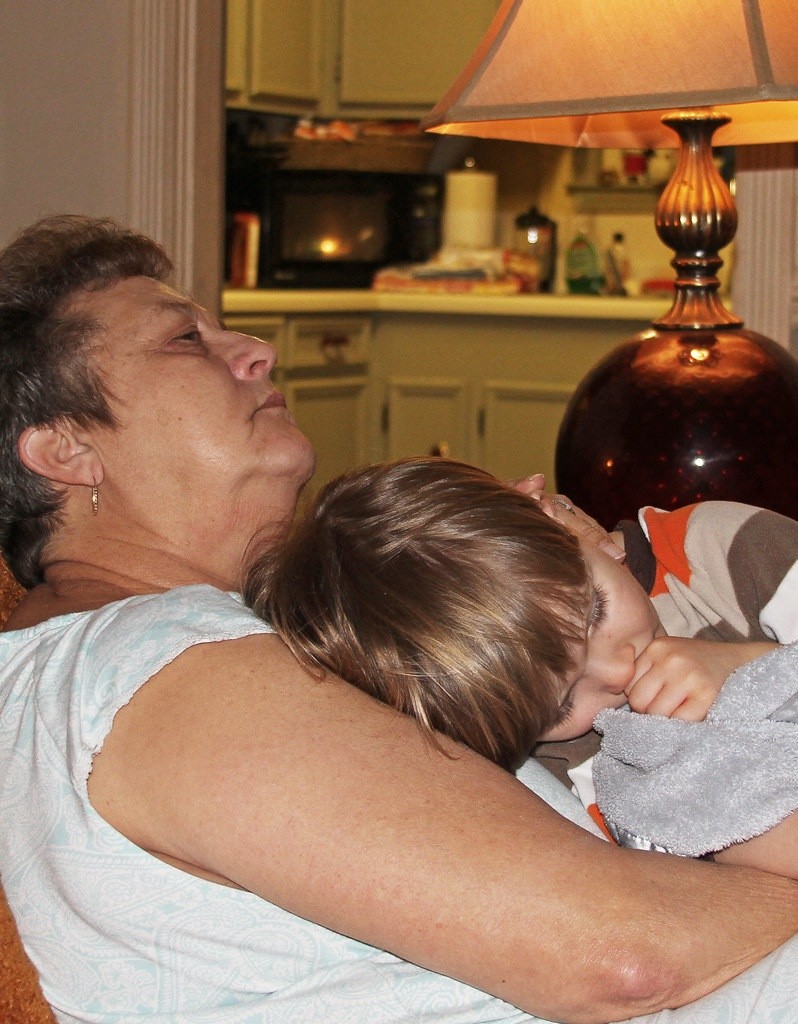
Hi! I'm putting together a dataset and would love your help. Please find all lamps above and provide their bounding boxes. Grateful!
[416,0,797,532]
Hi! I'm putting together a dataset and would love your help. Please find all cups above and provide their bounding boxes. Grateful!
[229,211,260,291]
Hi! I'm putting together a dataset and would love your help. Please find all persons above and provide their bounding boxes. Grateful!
[0,217,798,1022]
[243,461,798,880]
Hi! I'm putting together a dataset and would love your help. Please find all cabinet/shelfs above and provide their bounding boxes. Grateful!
[219,1,503,121]
[219,314,662,543]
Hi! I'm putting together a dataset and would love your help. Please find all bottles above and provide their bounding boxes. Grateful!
[564,224,604,299]
[605,232,630,296]
